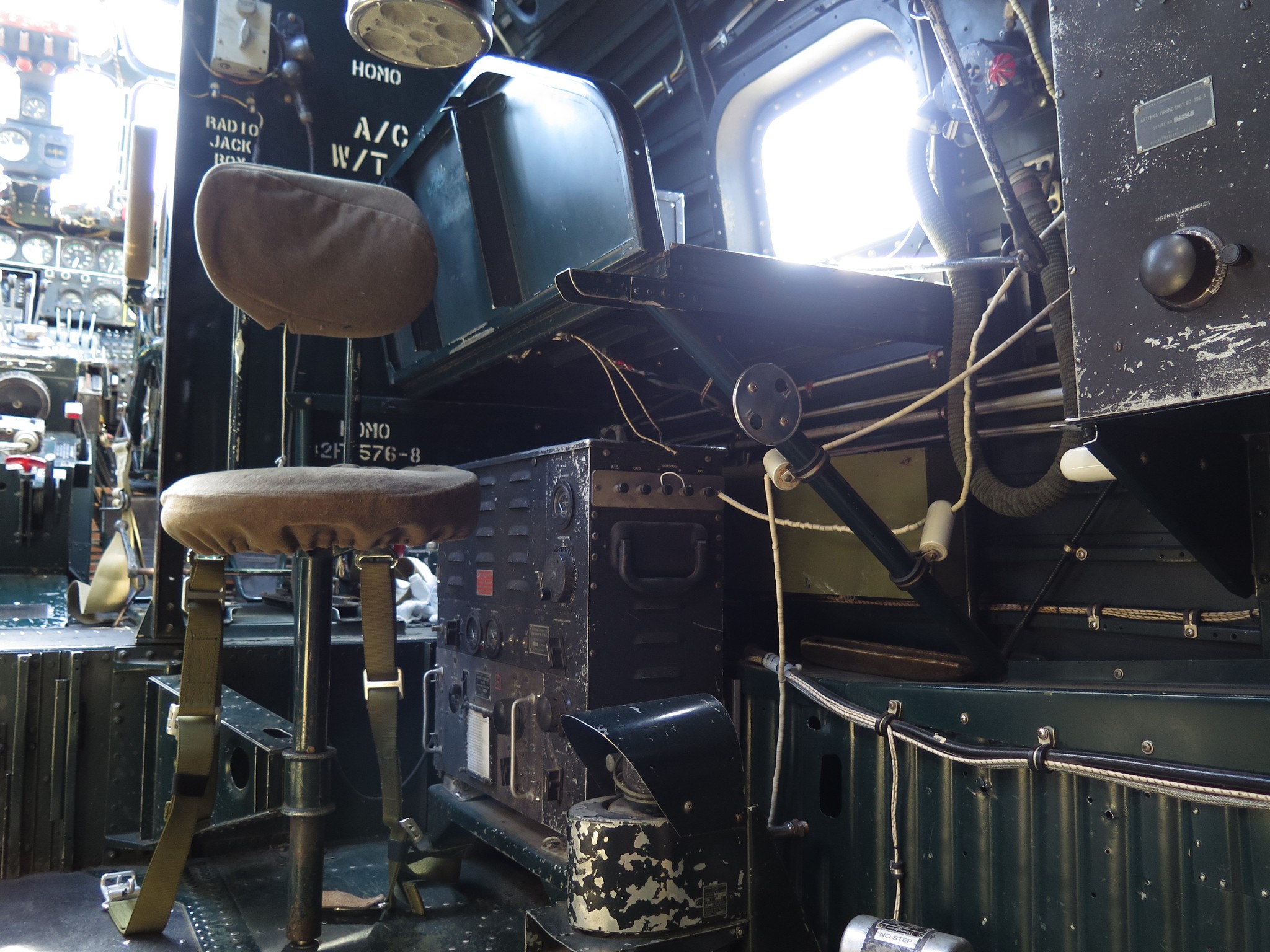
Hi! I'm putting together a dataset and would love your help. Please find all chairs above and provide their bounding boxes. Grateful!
[156,162,482,952]
